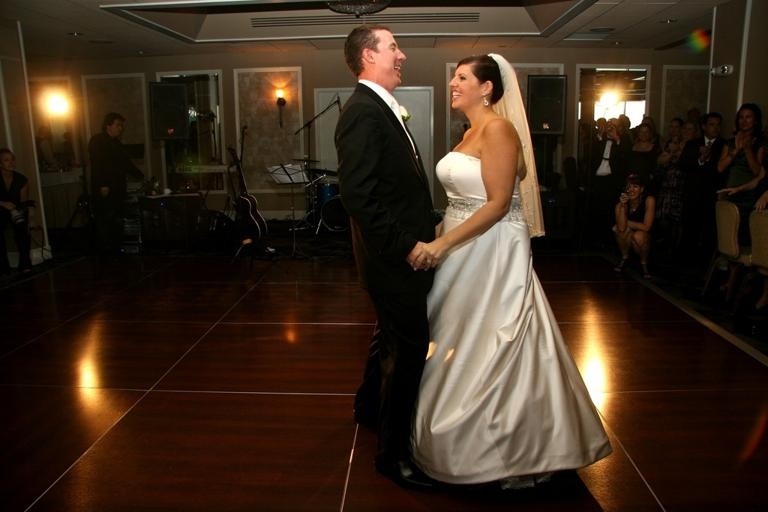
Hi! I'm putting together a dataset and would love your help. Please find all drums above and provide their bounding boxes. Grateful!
[321,194,351,232]
[317,181,340,209]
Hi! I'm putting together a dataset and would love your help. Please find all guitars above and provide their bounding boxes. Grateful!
[227,147,268,240]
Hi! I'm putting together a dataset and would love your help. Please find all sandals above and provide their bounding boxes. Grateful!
[639,260,652,279]
[613,254,630,272]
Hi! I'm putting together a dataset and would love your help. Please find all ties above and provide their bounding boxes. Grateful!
[706,141,713,154]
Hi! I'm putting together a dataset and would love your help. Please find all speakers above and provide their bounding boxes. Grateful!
[149,83,190,140]
[526,73,568,136]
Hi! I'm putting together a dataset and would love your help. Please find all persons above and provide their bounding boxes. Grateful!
[82,112,148,272]
[1,144,39,274]
[404,49,613,489]
[571,99,766,280]
[330,24,448,491]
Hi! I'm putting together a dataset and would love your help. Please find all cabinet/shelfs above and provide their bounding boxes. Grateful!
[139,195,201,257]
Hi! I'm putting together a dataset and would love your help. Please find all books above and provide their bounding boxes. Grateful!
[120,179,146,236]
[265,163,310,185]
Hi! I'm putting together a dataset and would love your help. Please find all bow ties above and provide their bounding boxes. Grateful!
[607,137,613,140]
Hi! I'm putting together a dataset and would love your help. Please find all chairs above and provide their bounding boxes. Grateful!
[702,198,768,319]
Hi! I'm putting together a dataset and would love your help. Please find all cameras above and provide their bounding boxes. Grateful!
[592,121,610,136]
[8,204,25,225]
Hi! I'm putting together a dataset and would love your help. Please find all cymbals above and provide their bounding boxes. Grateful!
[310,167,337,176]
[294,158,321,163]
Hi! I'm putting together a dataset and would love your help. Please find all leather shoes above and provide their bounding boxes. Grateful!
[501,471,554,490]
[376,450,437,489]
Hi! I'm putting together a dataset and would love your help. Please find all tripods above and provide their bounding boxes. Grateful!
[271,185,320,263]
